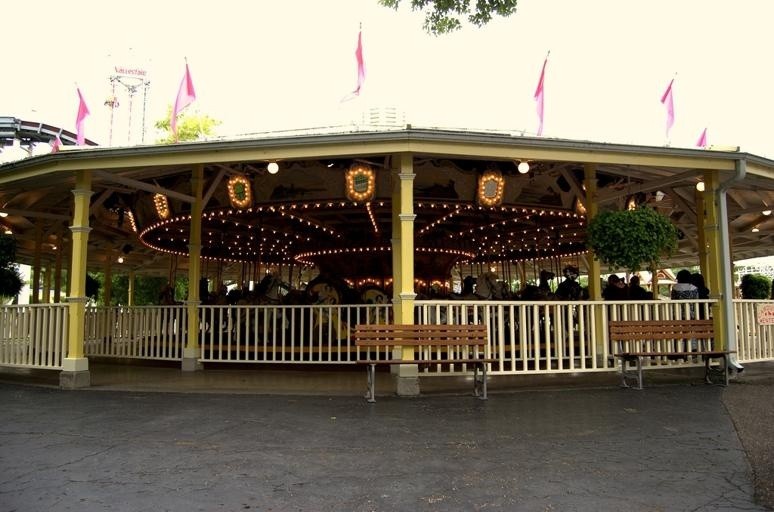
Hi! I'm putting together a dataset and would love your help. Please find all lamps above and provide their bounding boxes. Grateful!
[517,157,531,174]
[265,159,282,175]
[694,174,705,193]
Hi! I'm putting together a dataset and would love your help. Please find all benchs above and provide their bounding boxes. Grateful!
[607,315,741,391]
[350,323,500,403]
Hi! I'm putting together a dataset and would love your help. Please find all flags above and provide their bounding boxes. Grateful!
[696,128,707,147]
[172,64,197,129]
[352,31,366,97]
[51,135,62,153]
[661,78,675,130]
[75,88,90,145]
[533,60,547,135]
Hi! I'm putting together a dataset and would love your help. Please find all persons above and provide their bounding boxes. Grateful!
[603,268,710,322]
[156,267,583,341]
